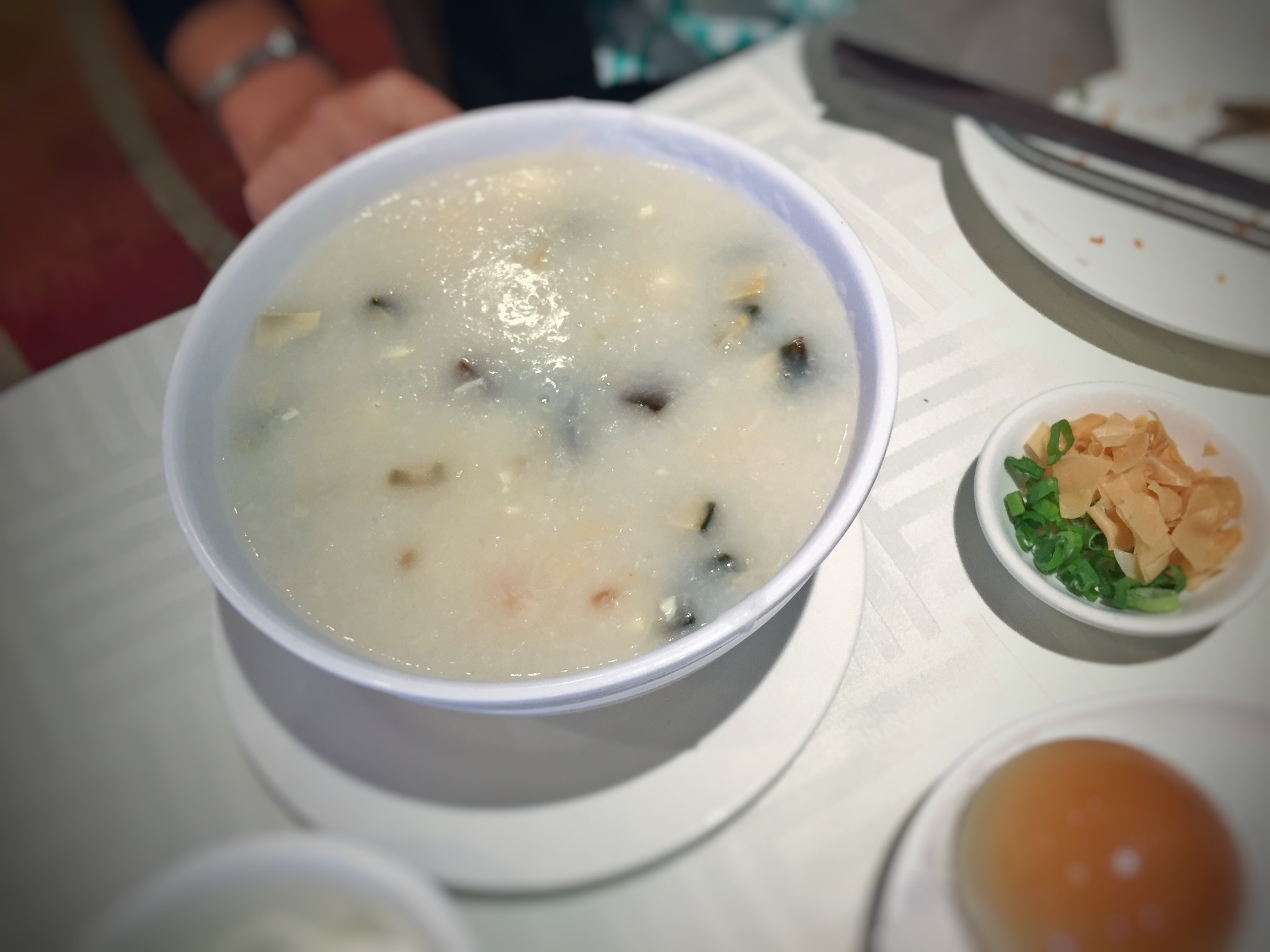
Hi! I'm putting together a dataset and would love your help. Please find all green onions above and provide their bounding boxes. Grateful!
[1005,418,1187,611]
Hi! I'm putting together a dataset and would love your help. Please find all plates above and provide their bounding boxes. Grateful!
[208,513,868,901]
[946,2,1269,356]
[65,832,476,952]
[864,697,1268,951]
[973,379,1269,640]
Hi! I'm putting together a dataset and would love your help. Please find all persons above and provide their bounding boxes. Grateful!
[122,0,464,231]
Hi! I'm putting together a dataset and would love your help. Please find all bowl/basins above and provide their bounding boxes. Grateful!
[159,100,900,719]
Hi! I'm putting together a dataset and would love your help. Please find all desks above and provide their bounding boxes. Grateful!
[1,33,1269,952]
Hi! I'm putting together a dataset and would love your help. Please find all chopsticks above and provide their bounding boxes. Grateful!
[833,43,1270,209]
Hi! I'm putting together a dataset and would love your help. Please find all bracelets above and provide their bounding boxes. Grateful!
[192,30,315,110]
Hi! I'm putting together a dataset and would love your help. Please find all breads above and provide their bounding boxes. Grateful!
[953,739,1242,952]
